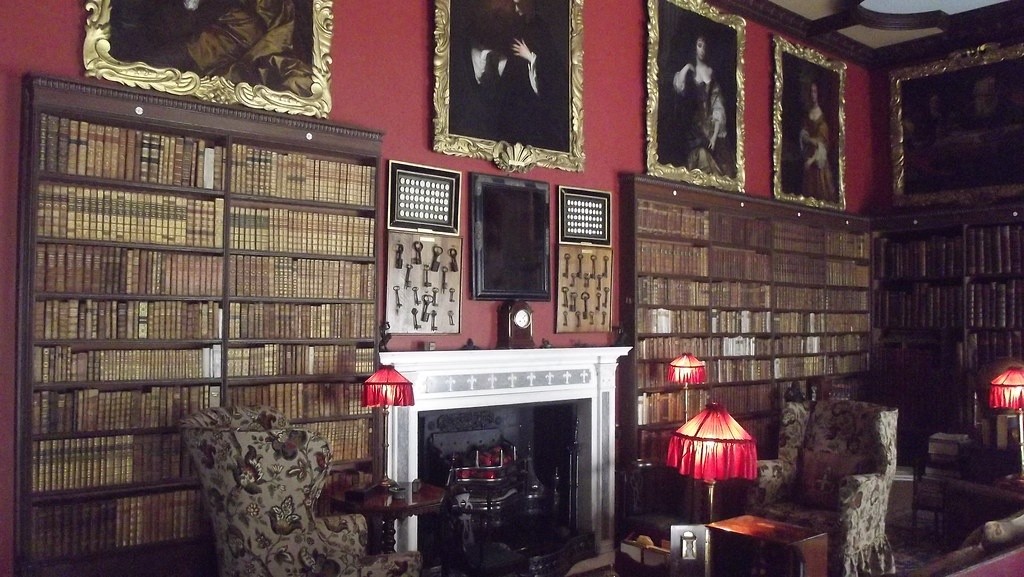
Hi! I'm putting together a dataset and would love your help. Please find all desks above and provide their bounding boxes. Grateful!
[945,469,1024,550]
[330,482,445,555]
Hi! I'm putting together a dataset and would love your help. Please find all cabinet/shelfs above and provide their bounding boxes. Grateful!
[15,72,385,577]
[616,172,1024,484]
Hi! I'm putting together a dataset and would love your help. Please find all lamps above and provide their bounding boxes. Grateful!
[361,365,415,492]
[668,353,707,424]
[666,403,758,577]
[989,367,1024,482]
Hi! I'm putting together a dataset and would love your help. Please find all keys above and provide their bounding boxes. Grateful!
[394,241,458,330]
[562,253,609,325]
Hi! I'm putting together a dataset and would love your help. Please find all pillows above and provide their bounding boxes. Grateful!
[793,449,860,512]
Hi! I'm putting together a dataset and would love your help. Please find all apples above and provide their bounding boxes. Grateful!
[458,445,512,479]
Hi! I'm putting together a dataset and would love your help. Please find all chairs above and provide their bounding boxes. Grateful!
[910,455,945,539]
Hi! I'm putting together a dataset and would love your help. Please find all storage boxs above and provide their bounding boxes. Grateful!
[704,515,828,577]
[928,433,968,455]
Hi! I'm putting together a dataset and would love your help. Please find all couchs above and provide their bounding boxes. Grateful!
[742,399,898,577]
[181,404,422,577]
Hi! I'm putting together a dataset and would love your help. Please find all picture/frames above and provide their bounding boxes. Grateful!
[647,0,746,194]
[468,172,552,303]
[433,0,585,173]
[387,160,462,237]
[889,42,1024,208]
[82,0,333,119]
[771,33,846,212]
[556,185,612,247]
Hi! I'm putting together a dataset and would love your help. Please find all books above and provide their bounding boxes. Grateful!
[38,114,376,261]
[33,243,377,340]
[28,419,370,560]
[31,344,375,436]
[641,335,872,466]
[636,276,871,333]
[872,223,1024,279]
[636,200,871,286]
[872,279,1024,530]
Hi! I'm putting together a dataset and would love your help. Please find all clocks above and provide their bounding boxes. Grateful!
[496,298,536,350]
